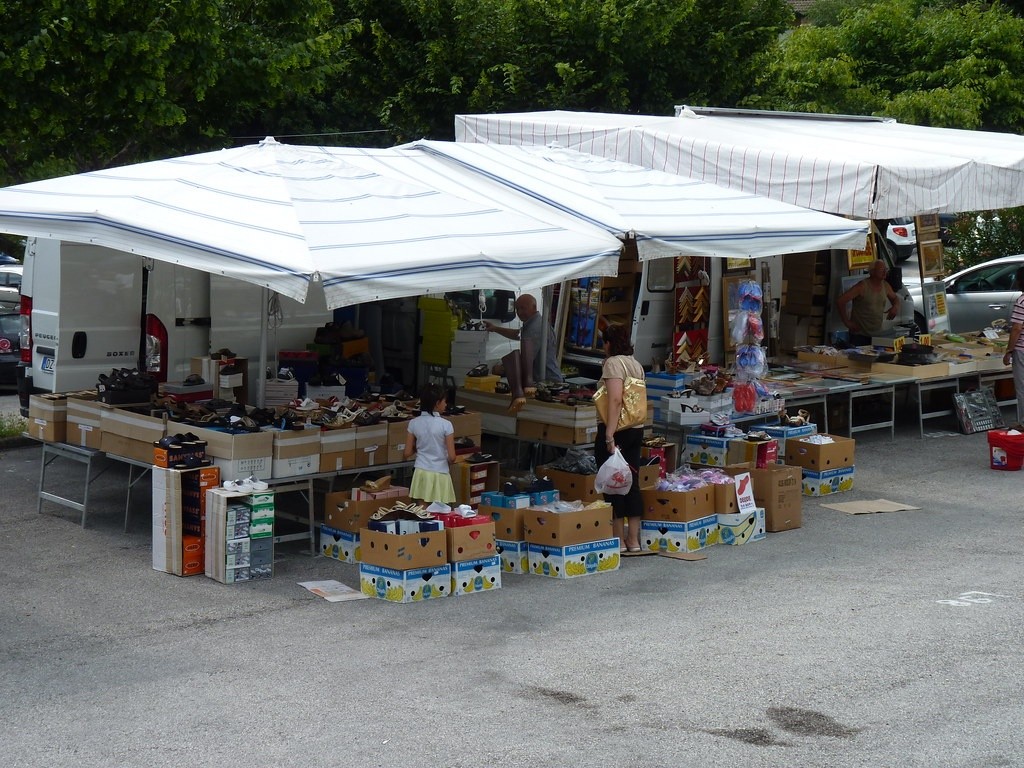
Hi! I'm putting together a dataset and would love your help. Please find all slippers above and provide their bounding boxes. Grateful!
[96,347,494,469]
[628,547,640,552]
[244,475,268,490]
[454,504,477,518]
[621,548,628,552]
[223,478,254,493]
[426,500,452,514]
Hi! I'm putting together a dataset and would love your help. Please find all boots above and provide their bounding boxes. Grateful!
[520,337,539,396]
[502,349,527,417]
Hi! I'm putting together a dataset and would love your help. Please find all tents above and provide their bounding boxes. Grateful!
[454,110,1024,220]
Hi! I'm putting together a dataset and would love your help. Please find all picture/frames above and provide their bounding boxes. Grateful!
[722,275,756,351]
[915,213,940,234]
[918,239,944,278]
[722,258,756,272]
[847,219,877,270]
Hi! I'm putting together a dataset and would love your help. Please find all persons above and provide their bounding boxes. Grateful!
[1002,266,1024,424]
[594,325,645,552]
[403,383,456,509]
[484,294,563,382]
[838,259,900,346]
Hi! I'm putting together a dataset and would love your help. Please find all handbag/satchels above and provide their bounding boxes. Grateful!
[592,356,647,433]
[594,447,633,496]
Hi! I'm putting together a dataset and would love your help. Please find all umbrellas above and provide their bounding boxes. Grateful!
[0,139,871,411]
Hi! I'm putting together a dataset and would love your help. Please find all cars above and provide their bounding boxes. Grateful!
[0,250,24,364]
[904,253,1024,339]
[878,212,972,265]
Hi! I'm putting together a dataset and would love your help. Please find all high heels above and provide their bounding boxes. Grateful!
[394,501,408,519]
[404,503,436,521]
[370,506,398,521]
[360,475,391,493]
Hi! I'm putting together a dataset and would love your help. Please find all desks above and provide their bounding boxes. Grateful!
[261,461,415,556]
[653,413,779,466]
[23,432,116,529]
[481,429,595,469]
[106,453,153,532]
[784,367,1017,439]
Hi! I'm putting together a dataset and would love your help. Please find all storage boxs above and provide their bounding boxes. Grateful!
[781,313,809,347]
[276,296,523,364]
[30,356,856,603]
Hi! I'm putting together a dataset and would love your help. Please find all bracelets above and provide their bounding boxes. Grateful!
[606,439,614,444]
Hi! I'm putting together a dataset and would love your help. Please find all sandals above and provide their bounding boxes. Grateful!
[491,279,811,514]
